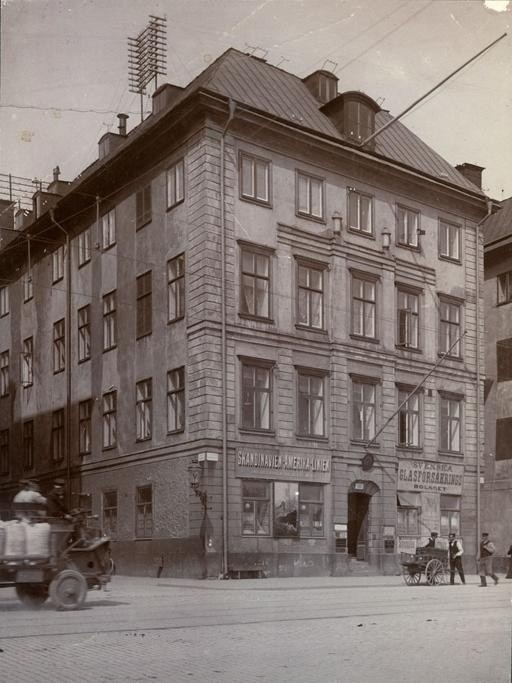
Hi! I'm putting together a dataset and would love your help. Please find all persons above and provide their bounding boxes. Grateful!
[449,534,466,584]
[48,477,88,547]
[14,477,49,522]
[477,533,499,587]
[421,531,438,547]
[506,546,512,578]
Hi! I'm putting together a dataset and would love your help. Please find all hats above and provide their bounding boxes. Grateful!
[54,478,66,489]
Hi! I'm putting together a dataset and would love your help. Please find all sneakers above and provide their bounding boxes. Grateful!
[449,580,465,585]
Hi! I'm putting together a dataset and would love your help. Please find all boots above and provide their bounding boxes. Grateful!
[478,574,498,587]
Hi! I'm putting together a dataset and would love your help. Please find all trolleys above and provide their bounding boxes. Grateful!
[401,546,461,587]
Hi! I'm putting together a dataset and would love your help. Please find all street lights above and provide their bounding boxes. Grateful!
[189,459,208,576]
